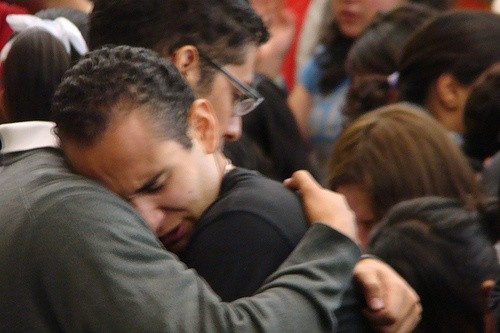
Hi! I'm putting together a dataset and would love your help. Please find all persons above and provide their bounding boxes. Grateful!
[1,0,500,333]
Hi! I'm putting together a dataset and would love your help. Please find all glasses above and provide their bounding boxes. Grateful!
[199,47,264,116]
